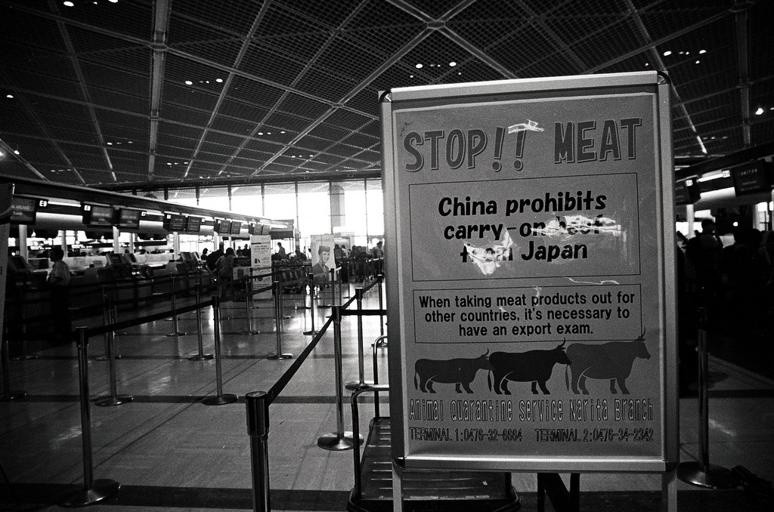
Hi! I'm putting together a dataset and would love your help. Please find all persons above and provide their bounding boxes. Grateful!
[124,248,132,265]
[47,247,74,360]
[202,241,384,300]
[86,264,97,272]
[167,248,175,261]
[685,218,722,265]
[6,253,33,360]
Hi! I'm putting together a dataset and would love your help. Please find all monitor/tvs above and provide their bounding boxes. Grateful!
[163,215,185,231]
[231,221,241,234]
[262,225,271,234]
[82,205,115,228]
[9,194,37,224]
[187,216,202,232]
[214,219,230,235]
[248,223,262,234]
[118,208,139,229]
